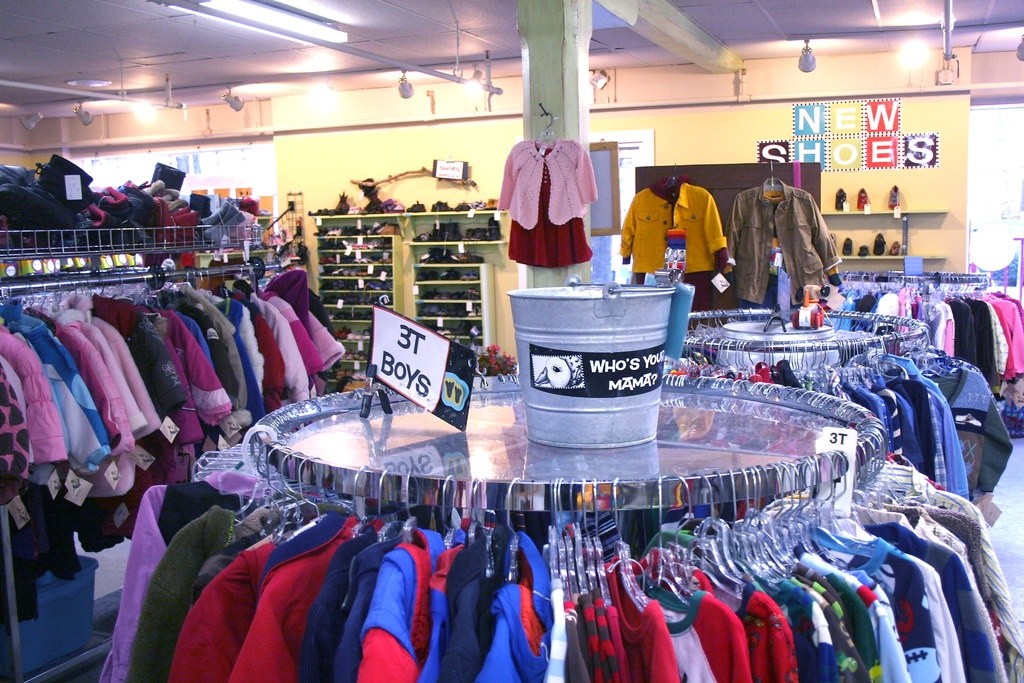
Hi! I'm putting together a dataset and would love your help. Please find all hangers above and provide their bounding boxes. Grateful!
[534,112,559,148]
[0,263,997,615]
[764,161,783,192]
[665,164,679,188]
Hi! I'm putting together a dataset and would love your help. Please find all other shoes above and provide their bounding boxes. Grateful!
[305,195,504,381]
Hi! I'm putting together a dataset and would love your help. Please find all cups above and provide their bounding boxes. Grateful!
[664,229,687,283]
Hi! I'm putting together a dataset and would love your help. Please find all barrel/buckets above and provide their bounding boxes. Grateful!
[507,274,676,449]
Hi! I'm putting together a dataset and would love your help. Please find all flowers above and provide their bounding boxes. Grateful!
[479,344,517,375]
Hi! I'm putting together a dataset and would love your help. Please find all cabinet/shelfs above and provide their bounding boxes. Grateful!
[309,214,511,373]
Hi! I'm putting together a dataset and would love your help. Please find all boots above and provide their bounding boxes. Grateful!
[0,153,253,250]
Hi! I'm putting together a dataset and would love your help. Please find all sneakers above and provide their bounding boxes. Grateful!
[889,241,900,255]
[835,188,846,210]
[842,237,852,256]
[873,233,886,255]
[857,188,868,210]
[888,185,899,210]
[858,245,869,256]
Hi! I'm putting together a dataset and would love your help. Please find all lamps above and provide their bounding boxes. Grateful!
[935,60,954,86]
[220,93,245,111]
[152,0,348,49]
[467,70,481,88]
[398,74,413,99]
[799,47,817,72]
[19,110,45,130]
[590,69,611,89]
[73,103,94,126]
